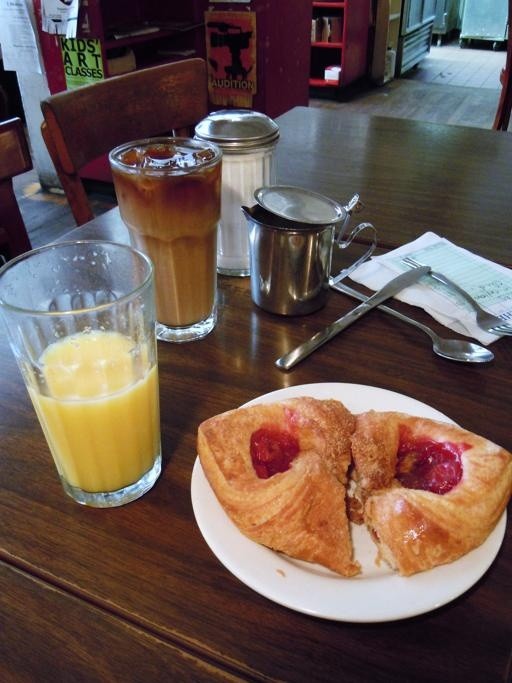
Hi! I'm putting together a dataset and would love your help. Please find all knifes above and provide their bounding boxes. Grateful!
[272,266,431,373]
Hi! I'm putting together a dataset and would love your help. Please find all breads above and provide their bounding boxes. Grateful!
[196,395,512,579]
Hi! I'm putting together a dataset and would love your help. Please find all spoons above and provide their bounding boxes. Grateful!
[330,275,497,364]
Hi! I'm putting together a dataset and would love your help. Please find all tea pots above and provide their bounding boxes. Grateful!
[239,183,381,319]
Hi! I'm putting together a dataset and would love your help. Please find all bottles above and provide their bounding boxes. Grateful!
[384,45,396,80]
[191,108,280,278]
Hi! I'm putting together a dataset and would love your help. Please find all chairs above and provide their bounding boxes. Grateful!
[41,58,209,226]
[0,117,34,268]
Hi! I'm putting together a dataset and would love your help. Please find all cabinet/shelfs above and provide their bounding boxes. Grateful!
[432,0,460,47]
[395,0,436,79]
[457,0,511,51]
[32,0,312,183]
[312,0,372,103]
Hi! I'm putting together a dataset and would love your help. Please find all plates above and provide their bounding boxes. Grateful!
[187,382,508,622]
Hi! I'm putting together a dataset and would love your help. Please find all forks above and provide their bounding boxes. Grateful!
[401,252,510,341]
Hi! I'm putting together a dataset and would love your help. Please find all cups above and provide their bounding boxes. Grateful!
[0,237,163,510]
[104,135,222,346]
